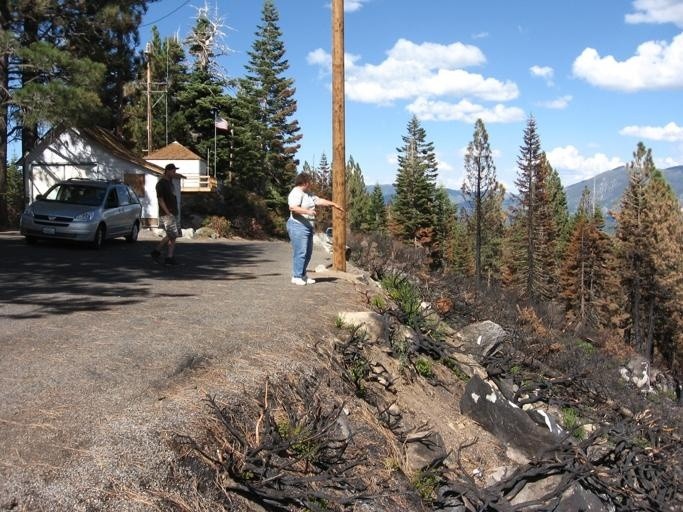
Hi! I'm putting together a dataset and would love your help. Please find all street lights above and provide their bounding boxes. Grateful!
[144,42,151,156]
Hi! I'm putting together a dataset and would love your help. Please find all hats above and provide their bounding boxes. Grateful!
[165,164,180,170]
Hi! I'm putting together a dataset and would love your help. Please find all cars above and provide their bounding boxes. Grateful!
[20,178,142,249]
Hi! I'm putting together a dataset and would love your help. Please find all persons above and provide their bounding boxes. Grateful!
[286,173,345,285]
[151,164,182,266]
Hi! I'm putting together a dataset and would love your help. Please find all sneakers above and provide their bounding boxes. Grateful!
[291,276,306,287]
[303,275,316,284]
[165,257,183,266]
[152,250,163,265]
